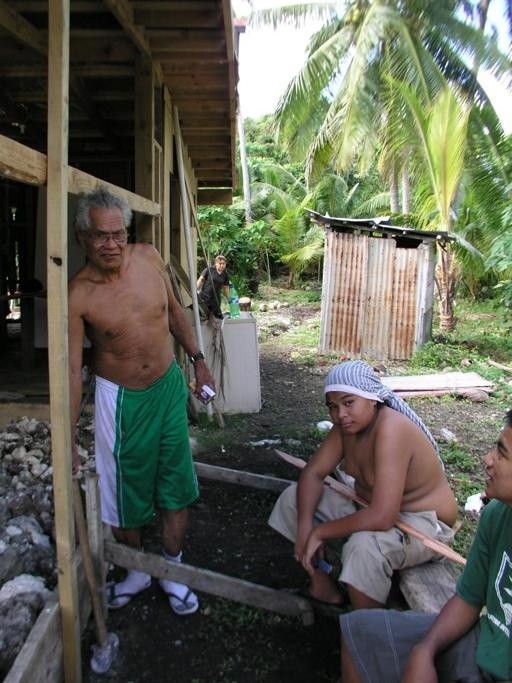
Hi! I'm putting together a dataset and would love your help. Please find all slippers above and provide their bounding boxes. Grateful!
[103,579,154,611]
[165,585,202,616]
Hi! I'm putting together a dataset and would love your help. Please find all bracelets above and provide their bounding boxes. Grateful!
[188,349,206,363]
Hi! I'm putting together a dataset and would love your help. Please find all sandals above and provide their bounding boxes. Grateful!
[278,582,349,616]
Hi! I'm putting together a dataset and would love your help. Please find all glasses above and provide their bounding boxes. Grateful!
[84,229,131,243]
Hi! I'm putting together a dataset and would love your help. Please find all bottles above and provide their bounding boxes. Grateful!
[228,283,240,319]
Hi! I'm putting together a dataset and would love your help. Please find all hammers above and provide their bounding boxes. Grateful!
[71,478,121,673]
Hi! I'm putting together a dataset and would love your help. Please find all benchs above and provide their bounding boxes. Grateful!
[400,561,456,614]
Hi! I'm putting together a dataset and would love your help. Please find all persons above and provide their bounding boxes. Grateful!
[61,179,218,622]
[263,355,462,621]
[196,254,232,321]
[336,407,510,682]
